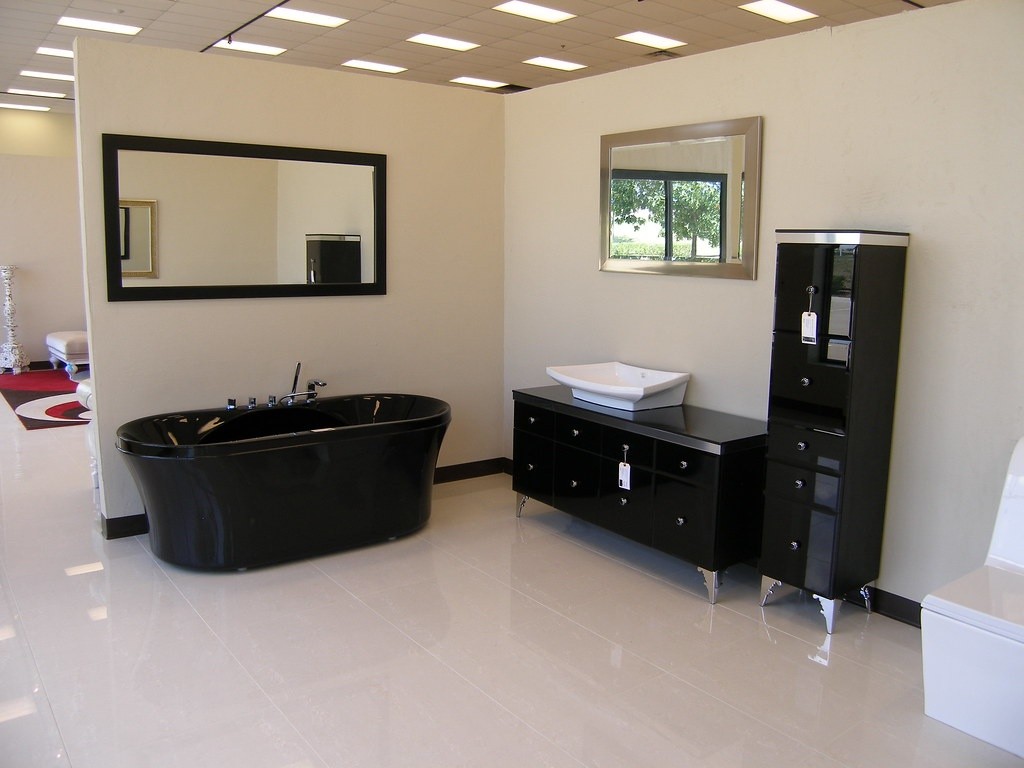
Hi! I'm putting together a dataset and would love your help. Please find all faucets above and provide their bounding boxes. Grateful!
[306,378,327,404]
[287,362,302,405]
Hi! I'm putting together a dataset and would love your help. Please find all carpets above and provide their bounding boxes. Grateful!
[0,367,91,431]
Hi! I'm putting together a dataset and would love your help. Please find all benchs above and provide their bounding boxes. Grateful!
[46,331,89,379]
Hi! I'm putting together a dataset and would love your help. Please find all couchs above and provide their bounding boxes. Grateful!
[76,380,101,522]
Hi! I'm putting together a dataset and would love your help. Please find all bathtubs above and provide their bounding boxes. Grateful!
[114,393,453,573]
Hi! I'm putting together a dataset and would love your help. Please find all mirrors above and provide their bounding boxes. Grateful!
[102,133,387,303]
[118,199,160,279]
[598,117,761,281]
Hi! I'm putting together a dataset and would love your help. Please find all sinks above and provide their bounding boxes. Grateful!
[545,361,690,412]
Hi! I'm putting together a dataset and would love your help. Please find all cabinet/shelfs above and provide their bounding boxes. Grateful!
[756,229,910,634]
[512,401,604,524]
[596,426,724,572]
[305,233,361,285]
